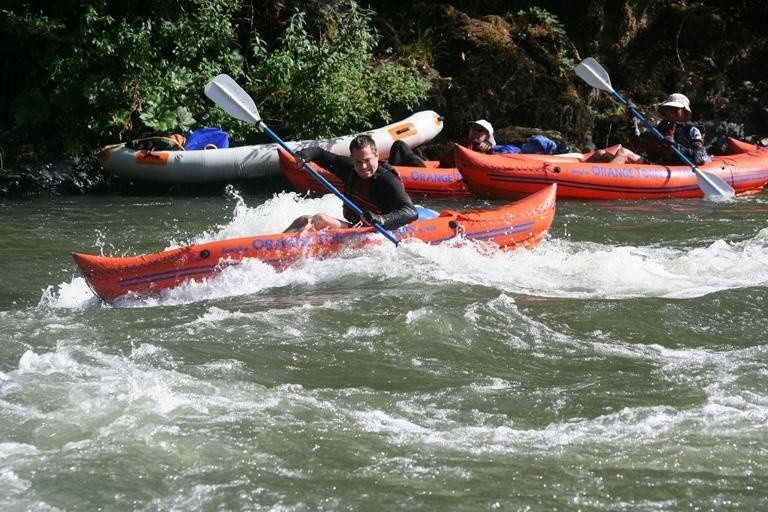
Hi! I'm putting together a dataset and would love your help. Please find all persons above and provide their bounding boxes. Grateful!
[389,118,496,168]
[582,93,710,165]
[282,134,422,236]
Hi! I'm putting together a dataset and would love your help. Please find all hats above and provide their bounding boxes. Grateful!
[661,93,691,112]
[467,119,494,136]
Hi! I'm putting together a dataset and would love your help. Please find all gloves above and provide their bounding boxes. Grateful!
[663,136,676,144]
[626,101,638,124]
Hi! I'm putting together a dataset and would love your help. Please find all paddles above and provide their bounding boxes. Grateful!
[575,57,735,203]
[204,73,401,246]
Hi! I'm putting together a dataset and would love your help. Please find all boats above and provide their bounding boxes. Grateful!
[97,111,444,188]
[455,138,766,201]
[281,145,642,198]
[70,180,554,302]
